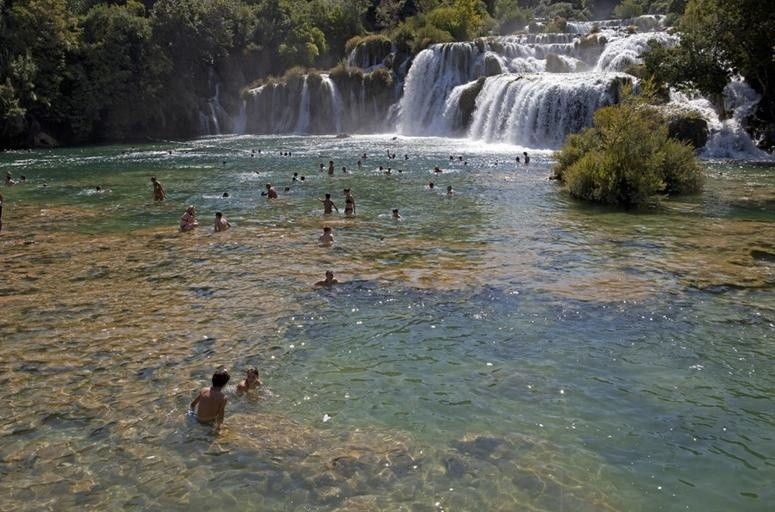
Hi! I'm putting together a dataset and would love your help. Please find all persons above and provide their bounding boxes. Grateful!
[319,226,334,242]
[392,208,401,218]
[318,163,326,172]
[3,169,17,186]
[190,367,231,433]
[214,211,232,233]
[523,151,529,164]
[343,188,355,216]
[342,166,348,173]
[150,177,167,202]
[318,193,338,215]
[179,205,199,232]
[328,160,334,175]
[236,365,263,401]
[264,183,278,199]
[446,185,456,194]
[386,149,395,159]
[434,167,442,172]
[313,269,337,288]
